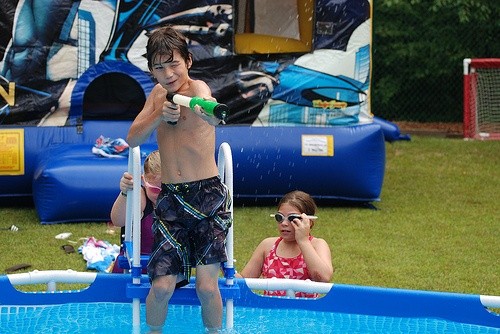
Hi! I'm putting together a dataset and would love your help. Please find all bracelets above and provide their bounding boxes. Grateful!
[121,192,128,197]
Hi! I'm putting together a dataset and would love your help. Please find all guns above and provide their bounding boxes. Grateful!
[164,91,227,125]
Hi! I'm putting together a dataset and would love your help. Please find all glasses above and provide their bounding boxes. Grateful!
[274,213,303,224]
[143,177,162,194]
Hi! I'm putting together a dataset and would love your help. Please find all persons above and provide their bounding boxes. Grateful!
[111,150,161,274]
[223,191,334,298]
[126,26,231,334]
